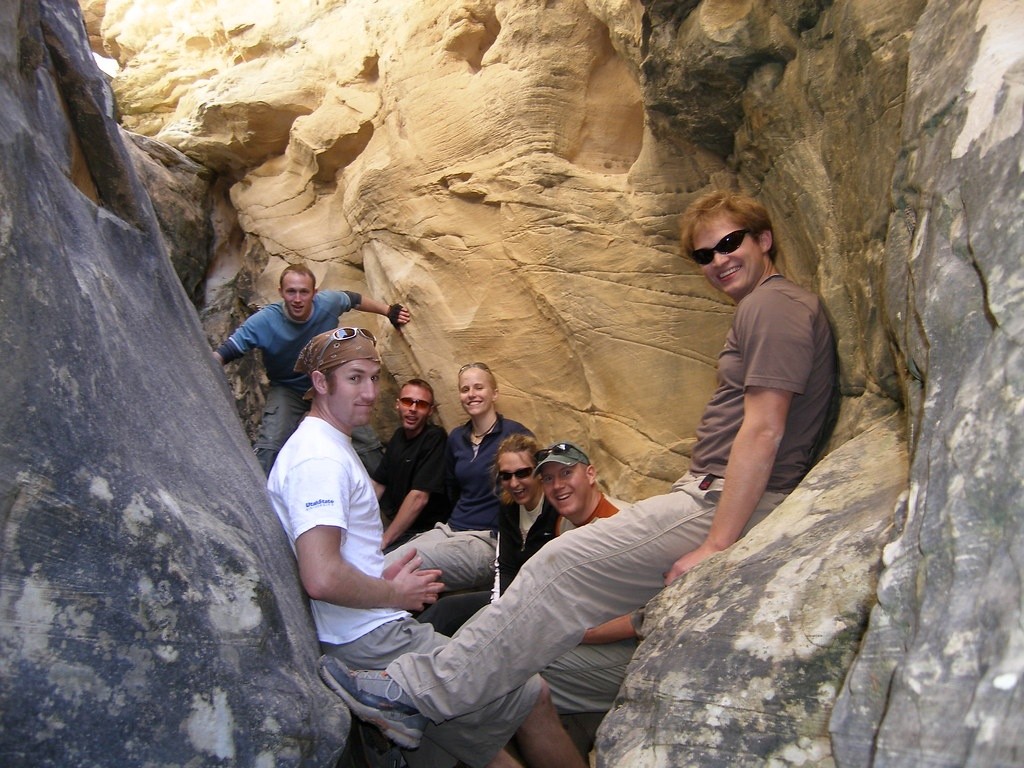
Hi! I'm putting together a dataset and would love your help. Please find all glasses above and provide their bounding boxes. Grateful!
[459,361,492,376]
[398,397,432,410]
[534,443,591,469]
[497,467,534,480]
[688,227,750,266]
[319,326,377,367]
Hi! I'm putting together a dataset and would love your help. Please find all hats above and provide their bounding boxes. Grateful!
[533,441,590,478]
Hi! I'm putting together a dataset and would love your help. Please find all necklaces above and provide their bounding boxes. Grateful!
[472,420,498,438]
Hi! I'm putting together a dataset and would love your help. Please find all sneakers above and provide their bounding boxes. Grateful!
[318,654,429,754]
[355,725,407,768]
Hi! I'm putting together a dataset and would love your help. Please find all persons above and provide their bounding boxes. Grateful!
[415,435,560,638]
[385,363,538,593]
[266,327,588,768]
[533,442,643,712]
[316,192,838,750]
[212,264,410,478]
[369,379,455,556]
